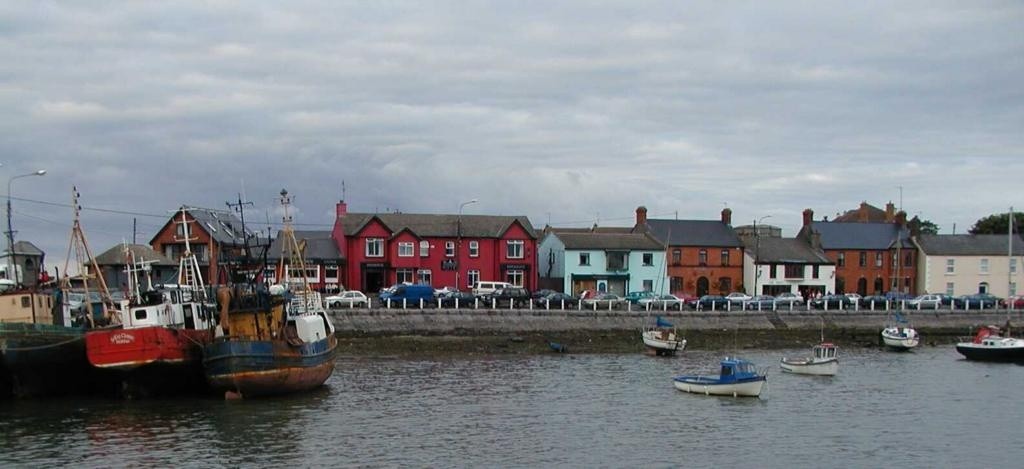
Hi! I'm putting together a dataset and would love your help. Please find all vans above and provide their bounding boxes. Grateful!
[470,281,512,298]
[379,285,434,307]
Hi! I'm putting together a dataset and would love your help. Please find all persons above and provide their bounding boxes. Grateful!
[38,271,49,285]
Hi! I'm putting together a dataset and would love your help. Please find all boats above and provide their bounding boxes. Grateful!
[201,185,341,396]
[779,343,840,376]
[83,202,218,395]
[0,317,124,401]
[744,287,1024,310]
[673,323,771,398]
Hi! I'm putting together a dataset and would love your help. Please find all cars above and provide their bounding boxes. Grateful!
[321,290,368,308]
[432,286,461,298]
[689,295,733,310]
[723,291,753,304]
[623,290,698,310]
[582,292,627,311]
[537,294,578,309]
[530,289,558,302]
[435,291,476,308]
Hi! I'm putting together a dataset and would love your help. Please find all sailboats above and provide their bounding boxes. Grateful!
[880,228,919,349]
[639,226,689,356]
[954,206,1024,361]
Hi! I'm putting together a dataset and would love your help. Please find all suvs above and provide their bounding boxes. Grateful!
[480,285,530,309]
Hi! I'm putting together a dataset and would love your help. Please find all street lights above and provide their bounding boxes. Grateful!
[754,214,773,296]
[8,170,48,291]
[456,199,478,288]
